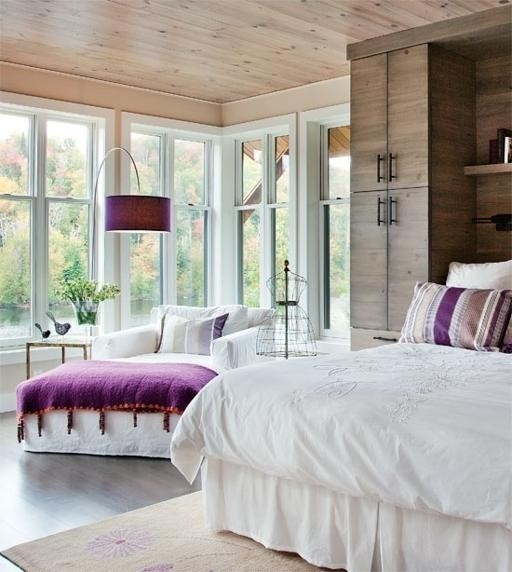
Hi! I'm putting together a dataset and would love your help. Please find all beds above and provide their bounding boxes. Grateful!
[172,344,511,572]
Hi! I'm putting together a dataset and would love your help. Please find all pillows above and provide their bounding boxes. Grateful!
[156,312,229,357]
[397,259,512,351]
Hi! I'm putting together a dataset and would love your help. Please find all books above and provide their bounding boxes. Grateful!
[489,128,512,164]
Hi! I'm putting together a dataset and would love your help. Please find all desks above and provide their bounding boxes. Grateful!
[26,335,93,379]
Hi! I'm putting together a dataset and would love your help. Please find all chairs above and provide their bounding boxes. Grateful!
[90,302,273,374]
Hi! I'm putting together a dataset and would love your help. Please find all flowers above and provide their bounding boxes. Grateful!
[52,261,120,323]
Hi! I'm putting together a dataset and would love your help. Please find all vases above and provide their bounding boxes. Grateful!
[71,301,99,324]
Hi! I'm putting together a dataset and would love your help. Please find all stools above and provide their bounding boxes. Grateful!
[16,361,218,460]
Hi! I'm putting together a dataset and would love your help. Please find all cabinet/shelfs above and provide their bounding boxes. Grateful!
[352,43,476,351]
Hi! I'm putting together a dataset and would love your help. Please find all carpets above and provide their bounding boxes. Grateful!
[1,489,343,571]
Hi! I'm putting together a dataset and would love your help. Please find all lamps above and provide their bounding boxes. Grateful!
[90,145,171,282]
